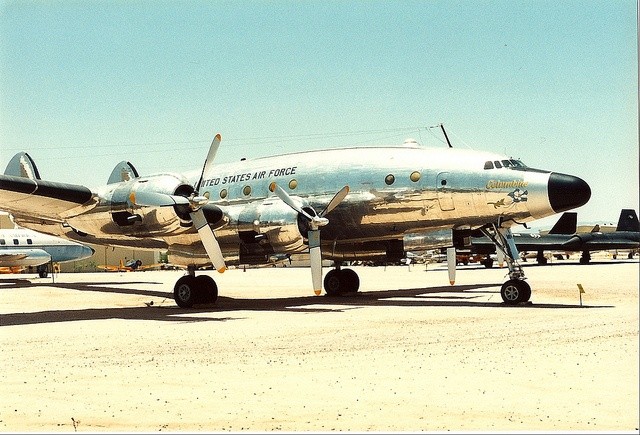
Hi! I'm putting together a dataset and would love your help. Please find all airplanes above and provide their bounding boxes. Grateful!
[97,258,165,272]
[0,228,96,278]
[440,211,577,268]
[0,134,592,307]
[561,209,640,264]
